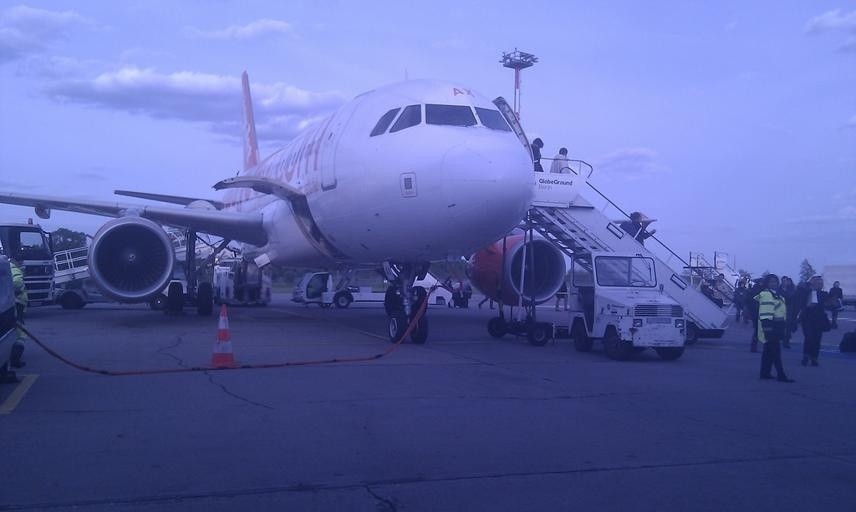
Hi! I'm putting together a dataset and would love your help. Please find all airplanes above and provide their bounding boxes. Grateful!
[0,73,581,354]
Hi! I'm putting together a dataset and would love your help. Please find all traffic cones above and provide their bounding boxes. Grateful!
[206,301,240,370]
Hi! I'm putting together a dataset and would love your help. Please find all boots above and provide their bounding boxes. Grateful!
[10,343,25,368]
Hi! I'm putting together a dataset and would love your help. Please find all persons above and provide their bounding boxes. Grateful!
[478,296,496,310]
[550,148,570,175]
[444,276,472,308]
[8,259,28,369]
[529,138,544,172]
[620,212,656,247]
[696,273,843,382]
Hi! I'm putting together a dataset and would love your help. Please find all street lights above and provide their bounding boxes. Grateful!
[497,46,540,130]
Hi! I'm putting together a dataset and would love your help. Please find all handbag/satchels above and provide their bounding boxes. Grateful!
[763,318,784,337]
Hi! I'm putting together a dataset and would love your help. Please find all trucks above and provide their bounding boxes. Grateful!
[0,221,58,310]
[804,262,856,311]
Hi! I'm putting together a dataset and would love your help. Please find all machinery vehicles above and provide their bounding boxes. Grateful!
[290,266,453,307]
[549,248,691,360]
[0,248,23,388]
[48,227,214,309]
[210,240,276,308]
